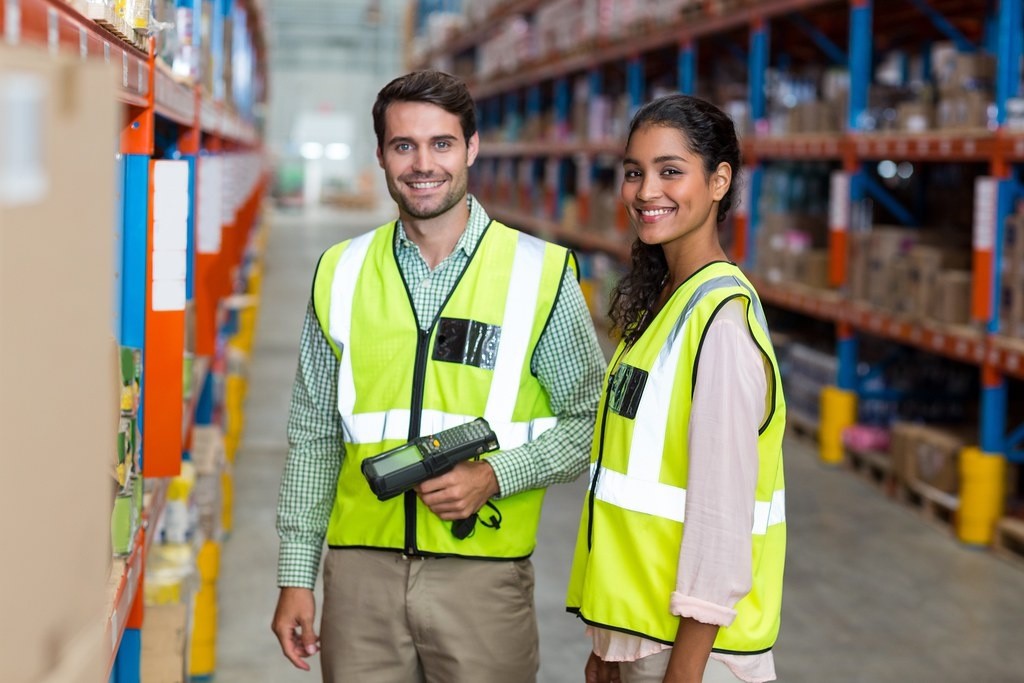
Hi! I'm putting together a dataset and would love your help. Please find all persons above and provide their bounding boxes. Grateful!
[566,96,786,683]
[271,69,608,683]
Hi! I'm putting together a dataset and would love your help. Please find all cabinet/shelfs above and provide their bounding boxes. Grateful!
[402,1,1024,548]
[1,1,268,683]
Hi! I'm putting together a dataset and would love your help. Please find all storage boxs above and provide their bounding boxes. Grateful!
[143,542,190,683]
[758,213,1023,341]
[892,417,977,493]
[792,56,995,135]
[2,42,118,683]
